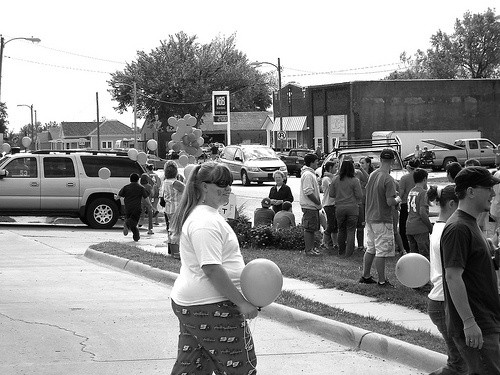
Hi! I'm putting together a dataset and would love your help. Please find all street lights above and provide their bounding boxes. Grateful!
[17,103,34,141]
[250,57,284,154]
[0,35,42,100]
[115,81,137,149]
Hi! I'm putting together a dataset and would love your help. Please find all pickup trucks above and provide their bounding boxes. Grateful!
[420,137,499,172]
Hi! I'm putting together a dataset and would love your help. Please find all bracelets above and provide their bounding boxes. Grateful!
[463,323,475,330]
[462,316,473,322]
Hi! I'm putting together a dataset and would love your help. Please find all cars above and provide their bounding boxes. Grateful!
[315,134,410,183]
[146,153,168,170]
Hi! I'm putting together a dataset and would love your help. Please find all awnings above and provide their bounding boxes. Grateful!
[273,116,308,132]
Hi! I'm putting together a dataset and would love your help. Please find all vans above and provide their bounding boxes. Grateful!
[0,147,157,230]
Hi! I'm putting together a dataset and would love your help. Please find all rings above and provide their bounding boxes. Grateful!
[470,340,472,343]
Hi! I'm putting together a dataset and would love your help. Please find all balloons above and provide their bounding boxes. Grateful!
[22,136,31,147]
[2,143,10,153]
[395,252,431,288]
[128,148,147,165]
[184,164,195,180]
[240,258,283,307]
[147,139,157,151]
[99,167,110,180]
[167,114,204,167]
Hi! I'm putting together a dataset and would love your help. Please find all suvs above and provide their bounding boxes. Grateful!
[281,148,315,178]
[218,144,288,184]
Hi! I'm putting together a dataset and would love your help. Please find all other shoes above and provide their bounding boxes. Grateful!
[377,280,395,288]
[133,231,140,241]
[359,276,377,284]
[304,247,320,257]
[123,222,128,236]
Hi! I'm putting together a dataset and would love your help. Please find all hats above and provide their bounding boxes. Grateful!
[380,149,394,159]
[454,166,500,190]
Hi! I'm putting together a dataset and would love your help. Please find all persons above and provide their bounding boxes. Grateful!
[299,148,500,296]
[415,144,429,163]
[253,170,296,230]
[440,166,500,375]
[114,162,186,242]
[427,184,460,375]
[210,144,217,160]
[171,162,257,375]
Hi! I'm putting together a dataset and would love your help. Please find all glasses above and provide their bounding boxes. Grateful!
[201,180,233,188]
